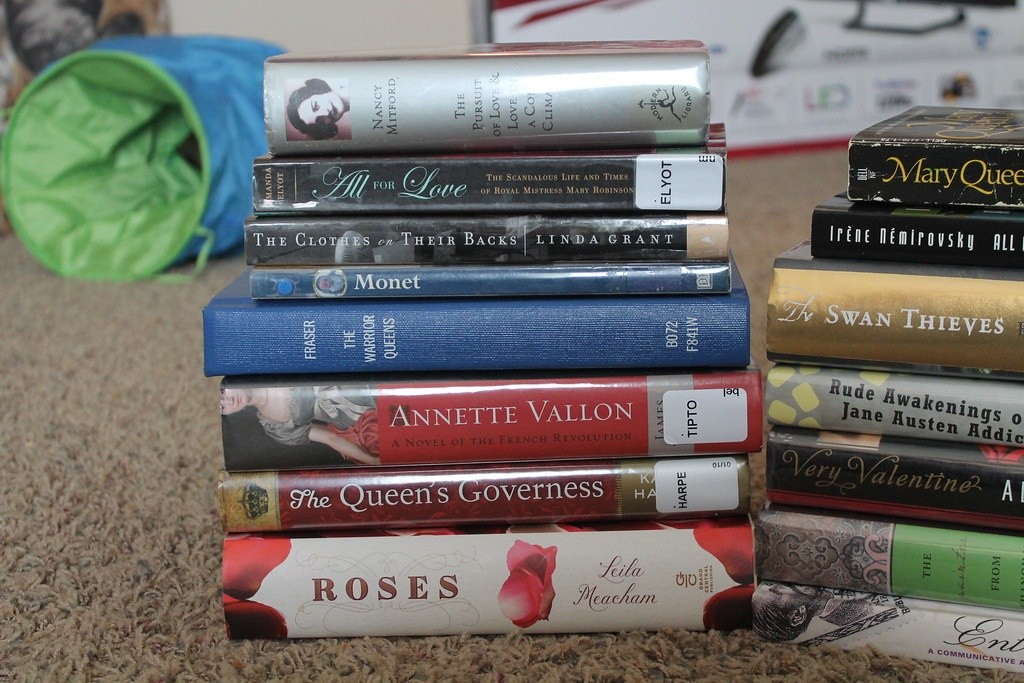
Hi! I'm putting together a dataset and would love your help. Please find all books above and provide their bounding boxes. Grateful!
[203,39,763,638]
[756,106,1024,671]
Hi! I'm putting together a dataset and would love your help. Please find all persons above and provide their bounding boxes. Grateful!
[287,79,351,138]
